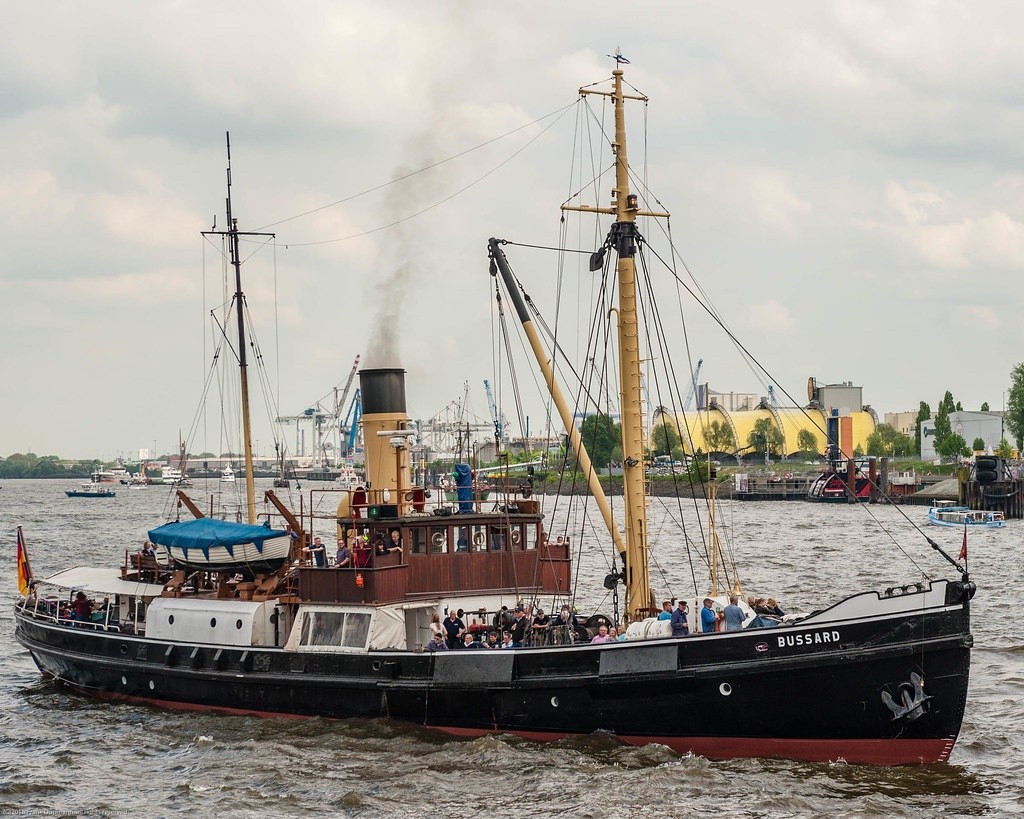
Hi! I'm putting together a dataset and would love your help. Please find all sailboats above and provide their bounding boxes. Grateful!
[11,44,977,765]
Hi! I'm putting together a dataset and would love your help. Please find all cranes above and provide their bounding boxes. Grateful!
[274,355,361,469]
[402,398,496,433]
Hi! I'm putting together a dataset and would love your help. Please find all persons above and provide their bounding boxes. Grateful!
[535,532,548,548]
[71,591,94,621]
[142,542,150,556]
[747,596,785,617]
[428,632,449,649]
[334,539,350,568]
[609,627,619,642]
[385,531,403,552]
[303,537,328,568]
[701,598,720,633]
[724,596,746,631]
[671,601,688,636]
[591,625,609,644]
[429,605,578,649]
[659,601,672,621]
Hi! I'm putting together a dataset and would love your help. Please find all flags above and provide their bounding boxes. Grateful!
[958,531,967,560]
[17,531,34,598]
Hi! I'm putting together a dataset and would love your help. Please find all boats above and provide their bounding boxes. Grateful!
[272,478,289,488]
[220,462,235,482]
[65,460,194,497]
[928,497,1006,530]
[330,470,368,490]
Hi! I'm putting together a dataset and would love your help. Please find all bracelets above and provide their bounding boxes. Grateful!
[339,564,341,566]
[310,549,311,552]
